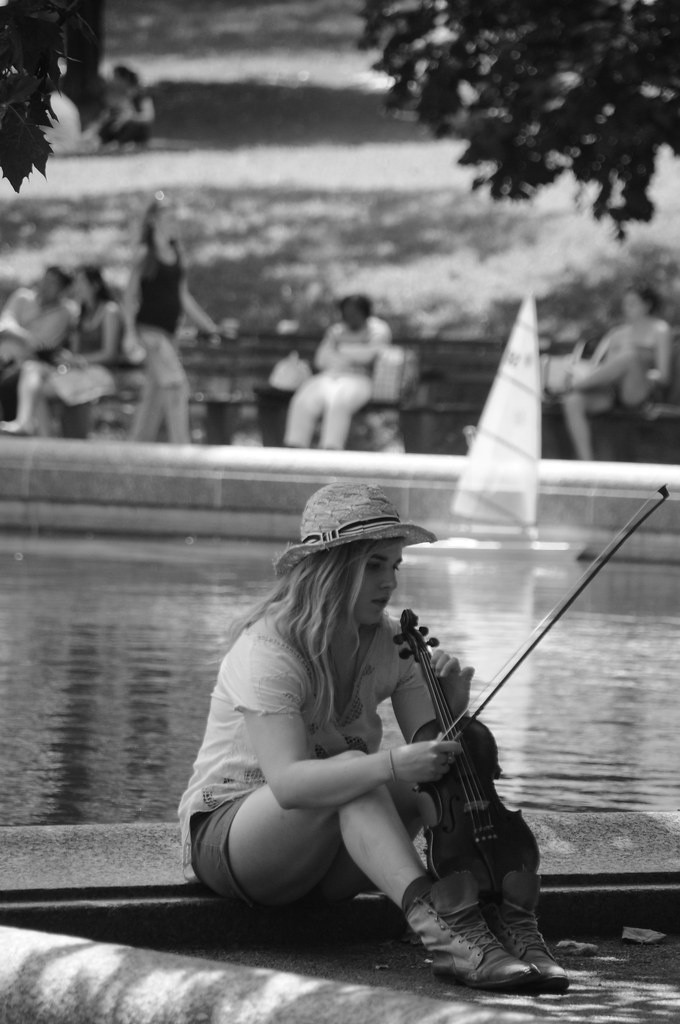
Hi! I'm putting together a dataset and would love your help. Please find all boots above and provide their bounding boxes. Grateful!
[402,870,570,992]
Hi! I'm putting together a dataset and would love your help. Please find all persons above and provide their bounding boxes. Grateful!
[0,262,122,434]
[96,65,158,154]
[283,292,391,449]
[1,265,80,382]
[547,283,672,460]
[123,197,220,443]
[177,478,572,991]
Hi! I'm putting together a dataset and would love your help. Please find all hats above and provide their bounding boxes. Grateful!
[274,479,437,580]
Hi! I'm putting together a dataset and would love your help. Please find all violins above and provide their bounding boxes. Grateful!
[393,607,541,895]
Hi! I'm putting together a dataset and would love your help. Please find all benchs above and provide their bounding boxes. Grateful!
[0,323,679,449]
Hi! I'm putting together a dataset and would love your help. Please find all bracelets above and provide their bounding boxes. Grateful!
[388,749,397,782]
